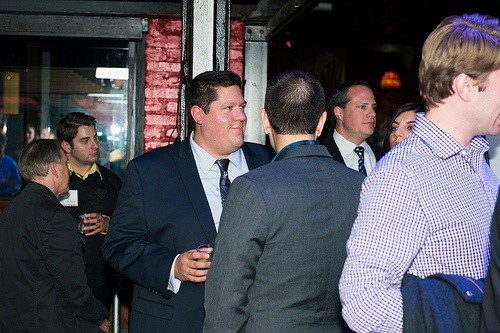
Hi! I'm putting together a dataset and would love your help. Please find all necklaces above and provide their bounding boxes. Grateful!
[271,139,319,165]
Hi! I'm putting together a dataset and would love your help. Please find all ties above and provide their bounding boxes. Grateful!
[216,159,230,211]
[354,146,368,179]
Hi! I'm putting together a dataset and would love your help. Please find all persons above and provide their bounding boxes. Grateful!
[203,70,365,332]
[374,119,391,149]
[57,111,122,313]
[0,138,111,333]
[101,70,277,333]
[317,79,388,177]
[338,11,499,333]
[383,103,428,153]
[0,111,129,201]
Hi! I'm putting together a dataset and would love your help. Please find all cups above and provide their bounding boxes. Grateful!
[77,215,92,234]
[197,243,215,270]
